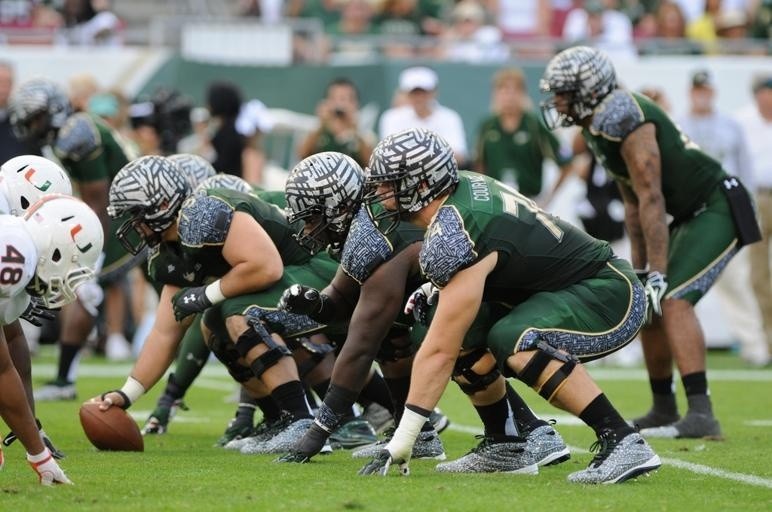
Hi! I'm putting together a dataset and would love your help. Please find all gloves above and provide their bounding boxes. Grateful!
[277,284,335,323]
[403,282,439,327]
[141,396,173,436]
[26,449,74,488]
[643,271,668,326]
[171,279,227,321]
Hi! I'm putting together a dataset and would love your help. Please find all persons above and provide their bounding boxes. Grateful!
[0,154,97,484]
[0,1,771,405]
[85,126,663,486]
[538,46,762,441]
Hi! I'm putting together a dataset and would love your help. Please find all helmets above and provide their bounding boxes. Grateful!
[0,155,104,287]
[539,45,618,122]
[7,76,70,140]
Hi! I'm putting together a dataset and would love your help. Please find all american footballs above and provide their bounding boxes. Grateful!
[79,402,144,451]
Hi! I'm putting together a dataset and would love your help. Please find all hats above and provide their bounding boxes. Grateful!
[400,66,437,94]
